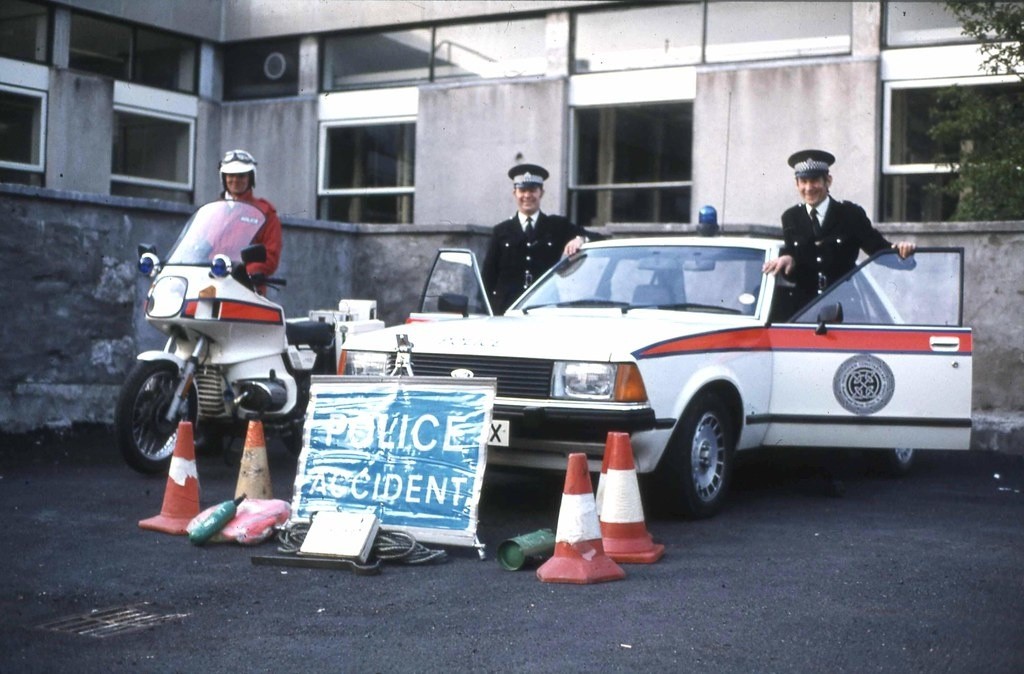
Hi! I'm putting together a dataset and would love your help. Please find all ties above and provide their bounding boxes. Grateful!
[525,217,534,242]
[810,208,821,235]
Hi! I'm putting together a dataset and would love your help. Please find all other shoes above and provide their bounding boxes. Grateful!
[194,435,225,456]
[822,476,845,497]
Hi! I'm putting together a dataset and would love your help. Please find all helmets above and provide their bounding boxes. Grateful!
[217,150,256,190]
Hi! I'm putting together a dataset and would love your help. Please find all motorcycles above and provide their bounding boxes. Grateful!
[114,198,333,477]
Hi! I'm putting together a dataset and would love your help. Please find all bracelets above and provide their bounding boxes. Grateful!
[577,235,585,243]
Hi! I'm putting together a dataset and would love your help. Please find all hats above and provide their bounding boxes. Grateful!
[788,150,835,178]
[508,164,549,188]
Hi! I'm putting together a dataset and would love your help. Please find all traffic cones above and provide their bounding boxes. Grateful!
[234,417,273,499]
[595,431,666,565]
[538,450,627,581]
[138,419,200,535]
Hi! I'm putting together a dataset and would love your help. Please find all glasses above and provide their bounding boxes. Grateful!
[223,152,252,163]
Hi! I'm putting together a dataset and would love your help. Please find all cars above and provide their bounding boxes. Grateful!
[338,204,975,516]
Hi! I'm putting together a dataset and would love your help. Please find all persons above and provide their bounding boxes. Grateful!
[762,150,916,496]
[476,165,586,314]
[195,149,282,457]
